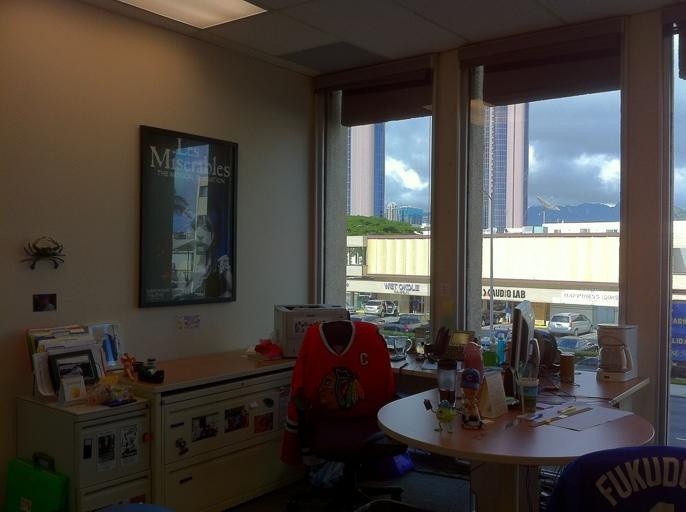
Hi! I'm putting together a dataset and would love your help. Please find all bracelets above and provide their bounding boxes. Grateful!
[224,288,232,294]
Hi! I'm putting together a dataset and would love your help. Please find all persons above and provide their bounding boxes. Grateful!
[192,405,249,441]
[37,293,55,311]
[505,304,513,324]
[461,342,484,419]
[121,425,137,457]
[209,234,232,300]
[411,297,419,314]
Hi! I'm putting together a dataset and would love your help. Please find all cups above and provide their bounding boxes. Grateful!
[394,337,412,356]
[437,359,457,407]
[518,378,538,414]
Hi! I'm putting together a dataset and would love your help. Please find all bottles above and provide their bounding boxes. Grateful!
[415,331,425,359]
[560,353,575,383]
[462,340,499,371]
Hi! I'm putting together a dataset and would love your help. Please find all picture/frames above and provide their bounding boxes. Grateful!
[139,126,238,309]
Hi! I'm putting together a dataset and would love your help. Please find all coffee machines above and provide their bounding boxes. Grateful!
[596,324,639,382]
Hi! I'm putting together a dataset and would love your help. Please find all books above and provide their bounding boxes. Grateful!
[23,320,124,402]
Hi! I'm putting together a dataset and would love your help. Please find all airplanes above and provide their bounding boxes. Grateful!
[536,197,560,216]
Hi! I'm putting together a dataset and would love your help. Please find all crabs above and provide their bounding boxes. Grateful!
[19,236,66,271]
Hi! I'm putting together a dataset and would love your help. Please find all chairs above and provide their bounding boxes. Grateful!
[299,320,408,510]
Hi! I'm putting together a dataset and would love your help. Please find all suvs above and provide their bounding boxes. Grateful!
[364,299,399,318]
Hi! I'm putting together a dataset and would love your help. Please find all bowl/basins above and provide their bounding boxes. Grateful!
[451,330,475,345]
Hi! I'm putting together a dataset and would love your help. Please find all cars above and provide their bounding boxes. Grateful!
[481,309,503,327]
[548,313,597,355]
[345,302,356,314]
[351,316,385,327]
[384,315,423,333]
